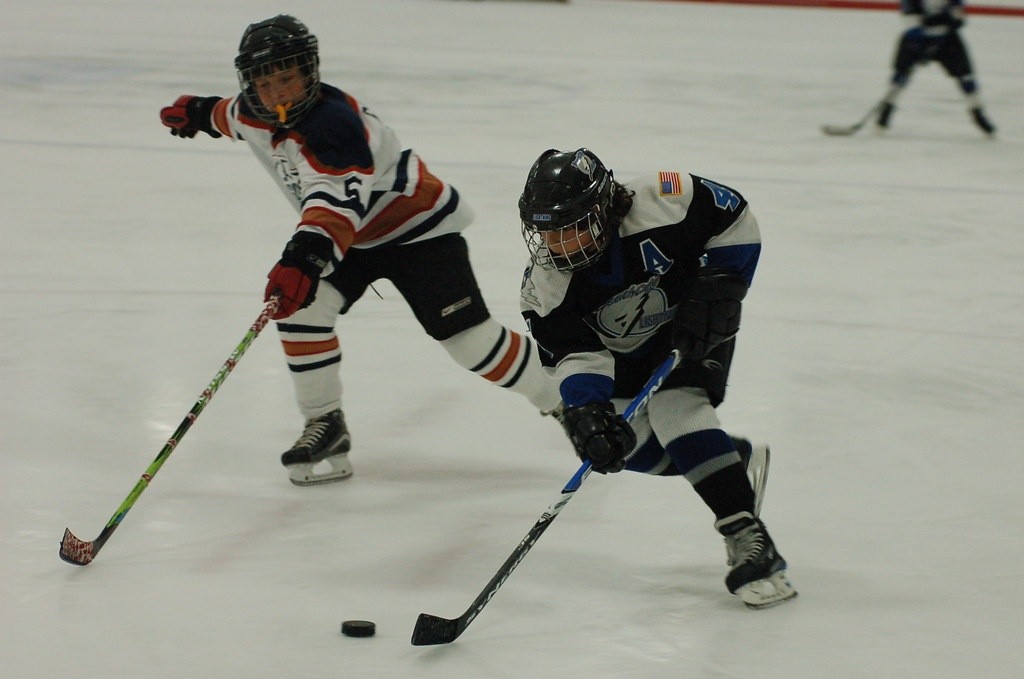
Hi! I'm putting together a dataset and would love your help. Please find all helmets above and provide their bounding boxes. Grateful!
[519,147,616,230]
[234,13,318,80]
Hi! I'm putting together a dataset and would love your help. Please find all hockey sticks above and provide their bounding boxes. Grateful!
[411,348,682,646]
[820,60,923,138]
[60,289,283,566]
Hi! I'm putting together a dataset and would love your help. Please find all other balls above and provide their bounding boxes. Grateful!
[341,620,375,637]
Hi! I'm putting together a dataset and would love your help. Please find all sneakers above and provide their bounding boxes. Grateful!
[875,101,896,133]
[714,511,800,612]
[726,435,771,519]
[971,112,999,139]
[282,408,355,486]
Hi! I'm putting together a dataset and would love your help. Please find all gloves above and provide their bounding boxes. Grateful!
[160,94,224,139]
[561,399,638,474]
[671,269,747,361]
[263,230,335,320]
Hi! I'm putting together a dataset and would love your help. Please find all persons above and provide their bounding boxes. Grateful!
[517,147,796,609]
[878,0,1000,134]
[159,15,573,484]
